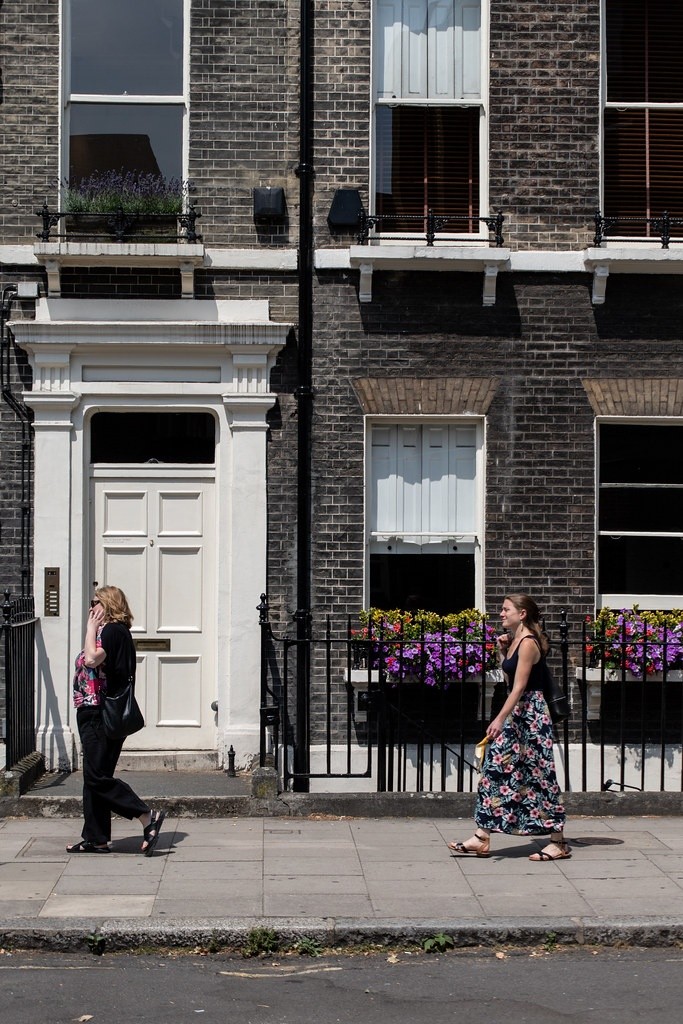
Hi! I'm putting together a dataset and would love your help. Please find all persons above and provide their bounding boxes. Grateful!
[65,585,164,856]
[448,593,572,861]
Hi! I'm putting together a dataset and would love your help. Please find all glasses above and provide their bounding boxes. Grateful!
[91,599,101,608]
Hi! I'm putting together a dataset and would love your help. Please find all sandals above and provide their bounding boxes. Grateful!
[449,833,490,857]
[65,839,113,853]
[140,807,164,856]
[529,840,571,860]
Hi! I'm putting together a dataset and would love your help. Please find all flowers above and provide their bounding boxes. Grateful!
[586,604,683,678]
[48,167,197,214]
[348,608,500,688]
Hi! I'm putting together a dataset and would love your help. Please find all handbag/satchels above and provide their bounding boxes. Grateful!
[544,666,570,723]
[102,681,145,738]
